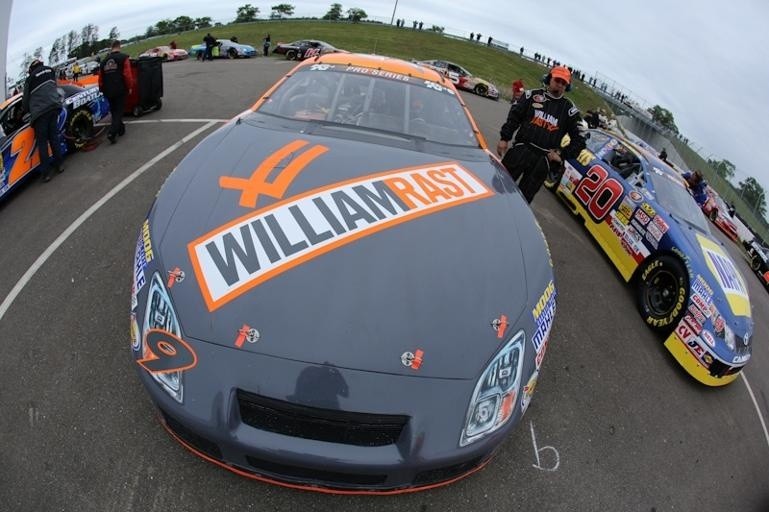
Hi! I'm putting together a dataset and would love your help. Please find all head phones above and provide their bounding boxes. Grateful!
[543,67,573,92]
[28,61,38,74]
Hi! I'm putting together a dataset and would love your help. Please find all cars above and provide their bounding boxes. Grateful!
[5,56,99,96]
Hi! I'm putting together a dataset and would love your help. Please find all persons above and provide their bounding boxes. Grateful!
[170,40,176,49]
[231,35,238,42]
[264,34,271,56]
[584,106,617,130]
[726,201,736,217]
[659,147,667,160]
[496,66,585,203]
[510,79,524,104]
[682,170,707,206]
[202,33,216,61]
[13,60,81,182]
[98,40,133,143]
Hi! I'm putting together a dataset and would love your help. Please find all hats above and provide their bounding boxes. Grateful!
[552,66,571,85]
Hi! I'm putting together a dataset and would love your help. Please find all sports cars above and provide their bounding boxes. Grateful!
[0,75,111,206]
[742,237,769,292]
[126,49,560,499]
[702,184,738,243]
[191,37,258,59]
[138,44,189,61]
[544,123,756,390]
[272,38,351,60]
[411,57,501,99]
